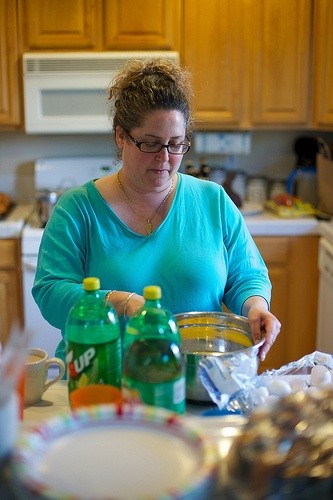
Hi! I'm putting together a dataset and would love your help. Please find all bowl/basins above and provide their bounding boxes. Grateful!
[173,311,266,402]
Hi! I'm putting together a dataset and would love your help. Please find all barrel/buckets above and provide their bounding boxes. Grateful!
[316,153,333,214]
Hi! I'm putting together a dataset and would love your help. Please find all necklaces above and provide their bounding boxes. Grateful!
[114,170,176,236]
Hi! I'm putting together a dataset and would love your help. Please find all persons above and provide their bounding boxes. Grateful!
[31,59,281,380]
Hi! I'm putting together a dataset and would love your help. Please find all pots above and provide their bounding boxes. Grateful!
[37,193,61,223]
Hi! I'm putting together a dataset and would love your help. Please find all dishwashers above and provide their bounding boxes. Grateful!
[315,237,333,355]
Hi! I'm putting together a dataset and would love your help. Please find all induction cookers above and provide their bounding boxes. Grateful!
[21,155,122,256]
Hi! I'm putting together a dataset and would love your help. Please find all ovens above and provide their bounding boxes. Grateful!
[21,256,64,380]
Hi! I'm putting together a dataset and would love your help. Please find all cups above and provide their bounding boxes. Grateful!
[21,347,65,404]
[287,166,318,208]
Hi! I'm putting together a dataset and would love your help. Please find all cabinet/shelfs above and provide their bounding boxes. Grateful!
[180,0,312,130]
[0,238,24,349]
[0,1,25,132]
[249,236,320,375]
[312,0,333,132]
[23,0,180,52]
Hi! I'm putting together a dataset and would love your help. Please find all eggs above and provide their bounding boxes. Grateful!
[254,365,331,402]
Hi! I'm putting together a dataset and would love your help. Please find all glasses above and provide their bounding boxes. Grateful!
[121,128,191,154]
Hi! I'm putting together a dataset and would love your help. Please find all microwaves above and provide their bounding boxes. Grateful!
[22,48,181,135]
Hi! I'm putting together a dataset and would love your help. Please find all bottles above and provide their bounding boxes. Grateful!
[185,159,194,175]
[121,285,185,416]
[66,277,122,412]
[199,157,210,180]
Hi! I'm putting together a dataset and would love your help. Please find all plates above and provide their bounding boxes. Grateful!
[239,201,263,215]
[10,401,217,500]
